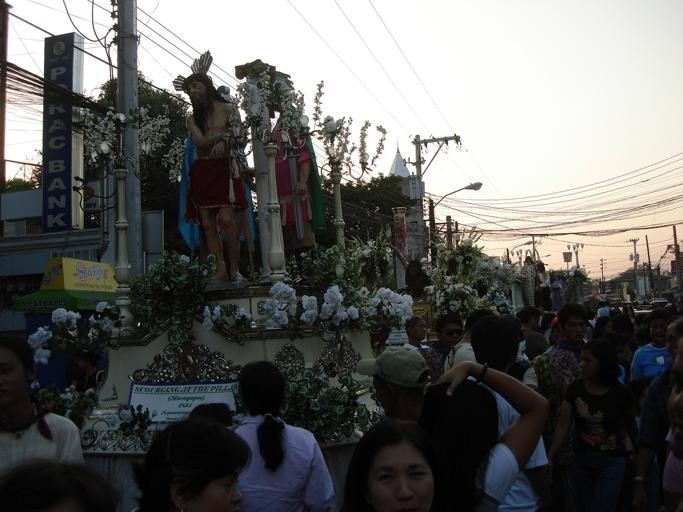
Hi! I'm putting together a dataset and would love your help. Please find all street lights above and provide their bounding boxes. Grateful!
[507,240,534,254]
[230,102,338,282]
[314,147,369,278]
[538,253,553,259]
[566,243,584,268]
[428,180,483,271]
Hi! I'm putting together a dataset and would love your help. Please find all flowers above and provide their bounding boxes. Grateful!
[24,70,513,445]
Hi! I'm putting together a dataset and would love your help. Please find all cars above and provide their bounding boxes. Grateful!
[608,293,671,318]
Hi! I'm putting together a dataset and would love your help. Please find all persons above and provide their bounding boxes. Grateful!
[549,271,557,284]
[187,400,233,430]
[182,72,251,285]
[535,262,552,311]
[521,256,536,274]
[0,454,121,511]
[138,416,253,512]
[553,274,566,290]
[0,335,84,477]
[339,301,683,512]
[271,110,317,263]
[230,357,336,512]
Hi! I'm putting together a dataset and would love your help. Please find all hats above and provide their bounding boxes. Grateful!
[356,344,431,388]
[550,317,558,331]
